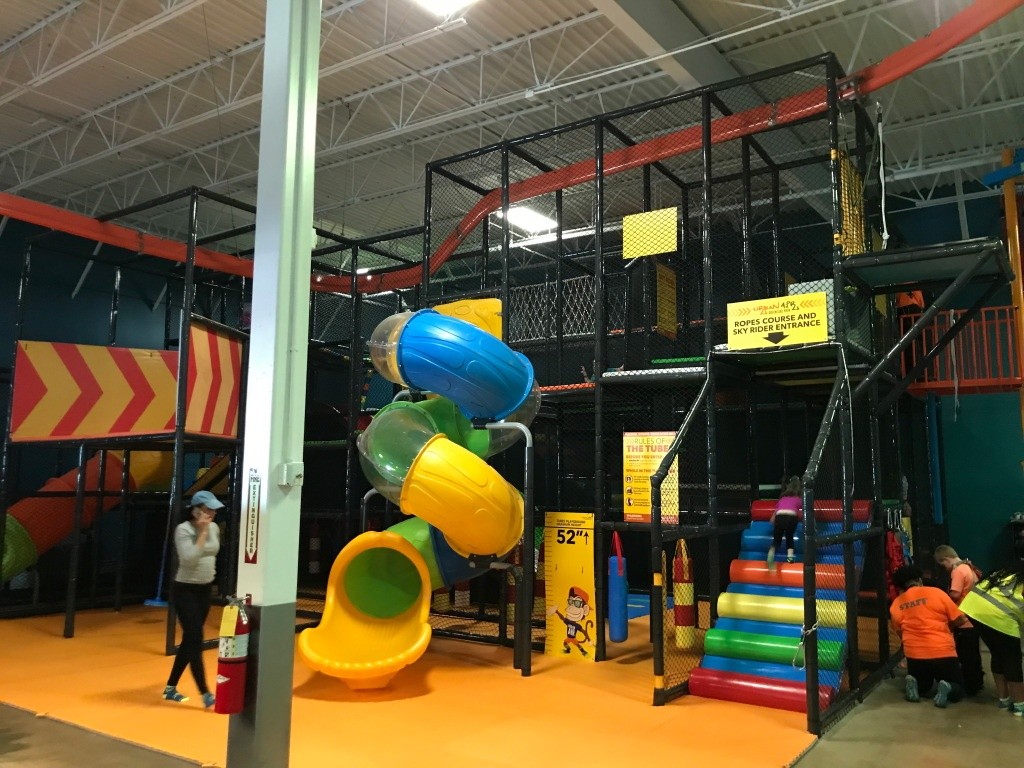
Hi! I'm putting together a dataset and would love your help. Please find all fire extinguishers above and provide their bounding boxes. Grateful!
[214,595,251,715]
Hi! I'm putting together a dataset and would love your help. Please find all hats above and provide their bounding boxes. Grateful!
[192,490,226,509]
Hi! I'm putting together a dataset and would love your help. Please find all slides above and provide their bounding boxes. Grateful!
[301,298,541,690]
[0,449,174,584]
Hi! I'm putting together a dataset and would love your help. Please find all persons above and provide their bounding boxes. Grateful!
[958,544,1024,715]
[897,288,932,383]
[935,543,987,698]
[160,490,225,708]
[534,433,559,508]
[765,474,804,569]
[889,563,969,707]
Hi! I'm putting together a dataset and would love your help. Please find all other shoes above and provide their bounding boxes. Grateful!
[904,675,920,702]
[933,679,951,709]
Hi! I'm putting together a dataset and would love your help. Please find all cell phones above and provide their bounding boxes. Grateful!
[192,507,210,523]
